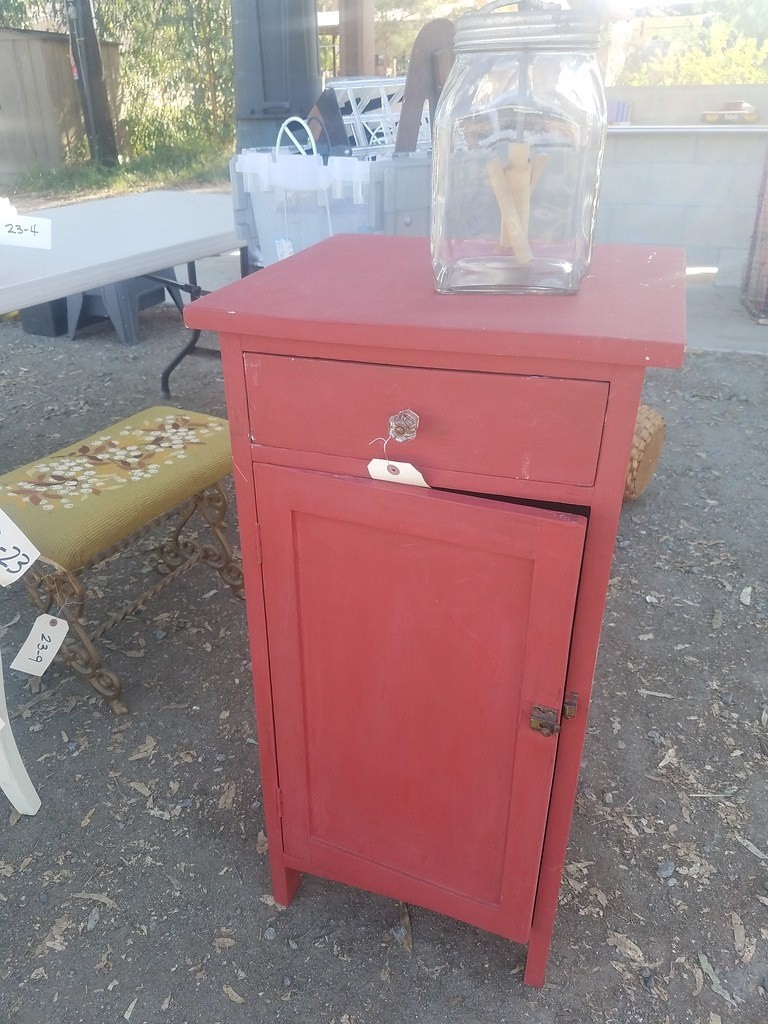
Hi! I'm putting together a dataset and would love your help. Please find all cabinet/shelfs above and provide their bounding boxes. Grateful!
[182,234,687,989]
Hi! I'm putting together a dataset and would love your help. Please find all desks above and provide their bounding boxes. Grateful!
[0,192,252,399]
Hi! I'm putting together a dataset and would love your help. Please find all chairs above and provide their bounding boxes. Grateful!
[65,267,185,346]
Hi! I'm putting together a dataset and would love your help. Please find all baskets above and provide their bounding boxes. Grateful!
[622,404,665,499]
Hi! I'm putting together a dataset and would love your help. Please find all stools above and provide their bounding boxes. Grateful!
[0,405,247,716]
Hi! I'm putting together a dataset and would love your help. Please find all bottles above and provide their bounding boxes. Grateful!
[431,9,608,298]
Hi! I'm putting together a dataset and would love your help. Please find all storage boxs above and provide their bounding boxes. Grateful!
[21,282,166,336]
